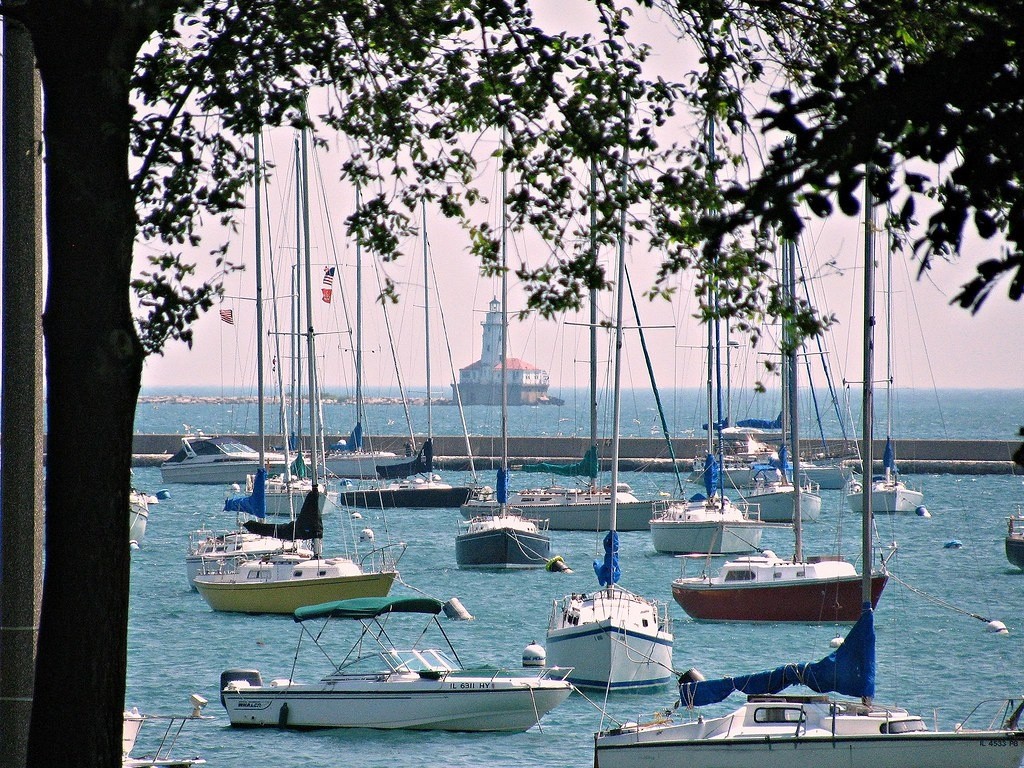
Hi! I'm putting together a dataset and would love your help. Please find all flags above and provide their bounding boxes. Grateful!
[322,267,335,285]
[321,289,332,303]
[219,309,234,324]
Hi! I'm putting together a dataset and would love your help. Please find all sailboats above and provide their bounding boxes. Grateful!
[182,78,926,622]
[592,165,1023,766]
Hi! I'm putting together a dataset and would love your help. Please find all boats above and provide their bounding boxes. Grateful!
[1004,505,1024,569]
[128,472,159,551]
[160,429,311,485]
[122,695,214,768]
[218,598,575,734]
